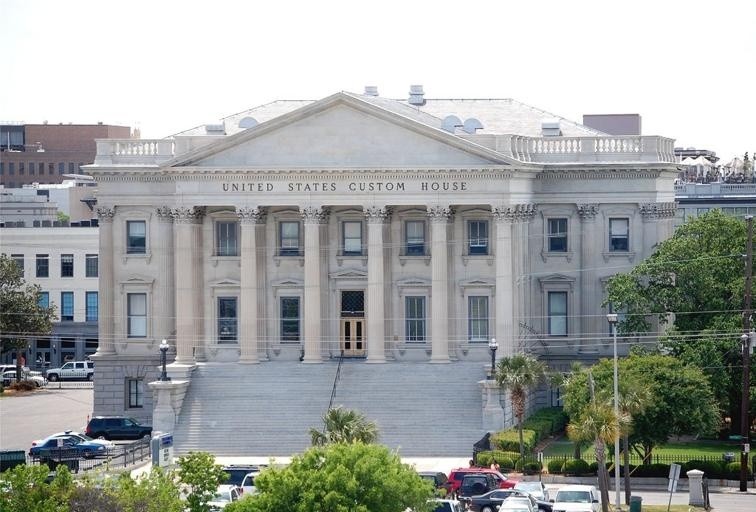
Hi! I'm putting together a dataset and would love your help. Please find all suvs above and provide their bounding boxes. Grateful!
[46,360,94,382]
[85,415,152,440]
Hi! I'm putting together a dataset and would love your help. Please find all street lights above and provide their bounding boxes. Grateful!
[487,338,499,379]
[604,313,626,511]
[159,338,170,381]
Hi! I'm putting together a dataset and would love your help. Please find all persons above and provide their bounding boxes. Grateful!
[468,459,476,467]
[490,458,501,472]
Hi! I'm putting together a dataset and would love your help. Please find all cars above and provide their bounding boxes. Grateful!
[0,364,48,388]
[28,430,114,461]
[415,466,601,512]
[200,462,269,511]
